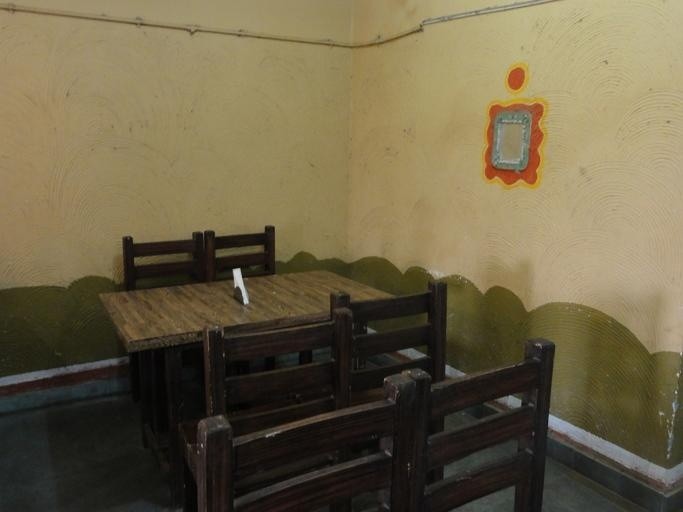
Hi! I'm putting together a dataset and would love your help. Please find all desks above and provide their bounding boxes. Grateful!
[103,269,398,427]
[97,268,401,490]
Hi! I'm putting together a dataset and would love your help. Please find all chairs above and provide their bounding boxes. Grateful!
[407,337,557,512]
[203,311,358,417]
[333,283,454,382]
[123,231,205,289]
[203,226,276,278]
[170,371,420,511]
